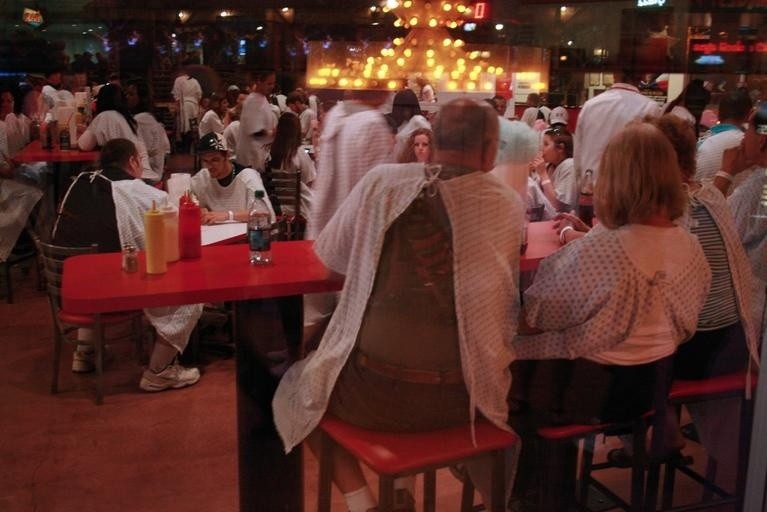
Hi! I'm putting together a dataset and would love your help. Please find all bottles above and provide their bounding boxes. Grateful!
[180,190,201,259]
[122,242,136,273]
[176,191,187,218]
[144,200,167,275]
[158,196,180,263]
[58,125,70,152]
[247,190,272,265]
[579,170,594,228]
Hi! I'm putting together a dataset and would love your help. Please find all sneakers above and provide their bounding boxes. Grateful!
[140,356,200,390]
[72,345,113,371]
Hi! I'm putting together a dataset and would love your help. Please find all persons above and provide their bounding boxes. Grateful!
[0,59,767,324]
[605,112,763,470]
[273,91,529,509]
[42,137,201,394]
[512,123,715,509]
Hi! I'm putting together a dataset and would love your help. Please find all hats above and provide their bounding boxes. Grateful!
[195,132,226,153]
[550,106,568,125]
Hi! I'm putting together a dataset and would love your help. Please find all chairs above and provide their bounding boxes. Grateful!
[163,107,301,239]
